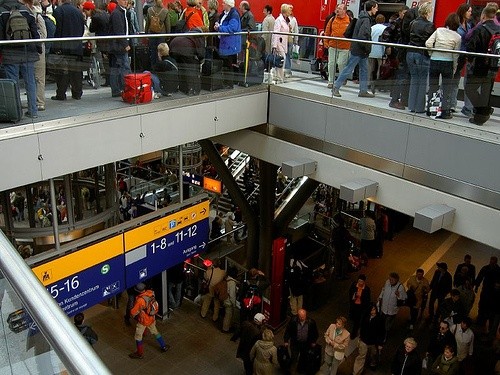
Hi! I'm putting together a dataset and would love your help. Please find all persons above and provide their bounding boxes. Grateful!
[0,0,499,126]
[8,142,500,375]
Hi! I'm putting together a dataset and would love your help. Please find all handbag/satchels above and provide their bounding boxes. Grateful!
[97,13,130,55]
[263,48,284,68]
[343,18,358,39]
[198,268,214,294]
[395,283,410,307]
[277,345,292,375]
[334,349,345,361]
[291,44,301,65]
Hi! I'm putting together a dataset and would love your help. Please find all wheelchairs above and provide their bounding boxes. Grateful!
[81,38,100,89]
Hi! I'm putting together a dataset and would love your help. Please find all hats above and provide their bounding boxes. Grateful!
[254,312,265,325]
[134,283,146,292]
[436,262,447,270]
[82,1,117,13]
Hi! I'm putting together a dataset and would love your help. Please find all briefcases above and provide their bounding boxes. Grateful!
[44,49,79,76]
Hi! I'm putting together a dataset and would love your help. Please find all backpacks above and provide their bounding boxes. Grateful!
[478,23,500,71]
[148,7,166,33]
[34,11,56,48]
[0,3,35,48]
[122,72,153,105]
[139,295,159,316]
[215,275,239,301]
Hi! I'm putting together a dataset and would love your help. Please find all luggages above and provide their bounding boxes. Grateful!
[305,344,322,375]
[0,78,24,124]
[177,35,265,97]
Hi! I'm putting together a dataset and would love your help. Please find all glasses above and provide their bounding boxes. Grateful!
[439,326,448,330]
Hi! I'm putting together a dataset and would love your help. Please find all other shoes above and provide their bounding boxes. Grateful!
[388,97,494,126]
[327,84,379,98]
[24,105,45,119]
[128,344,175,359]
[51,93,82,101]
